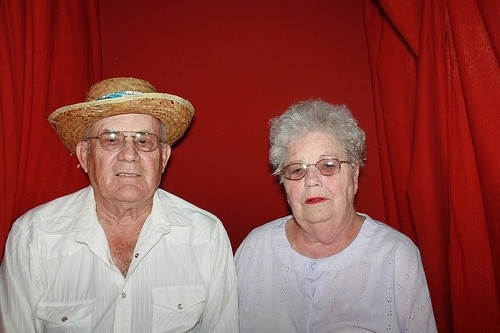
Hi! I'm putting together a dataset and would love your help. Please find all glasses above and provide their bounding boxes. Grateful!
[86,131,162,152]
[282,159,349,180]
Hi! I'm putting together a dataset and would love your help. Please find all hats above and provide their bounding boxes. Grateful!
[48,78,194,156]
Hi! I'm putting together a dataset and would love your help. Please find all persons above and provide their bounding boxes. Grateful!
[0,75,243,333]
[232,99,440,333]
